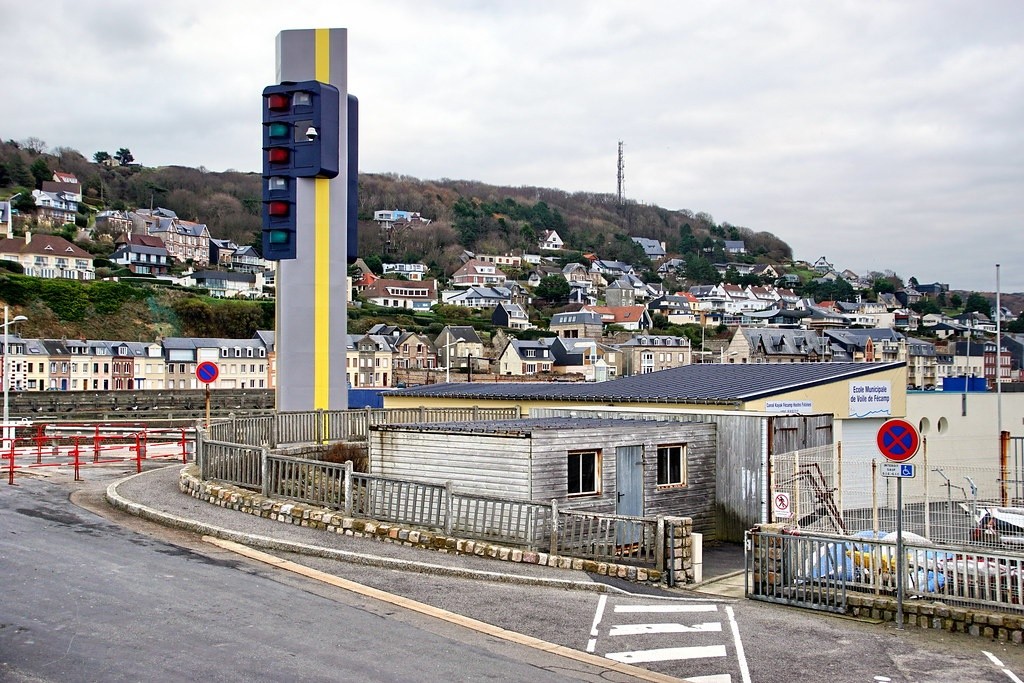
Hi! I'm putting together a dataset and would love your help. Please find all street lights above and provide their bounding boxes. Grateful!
[1,306,28,448]
[442,333,465,383]
